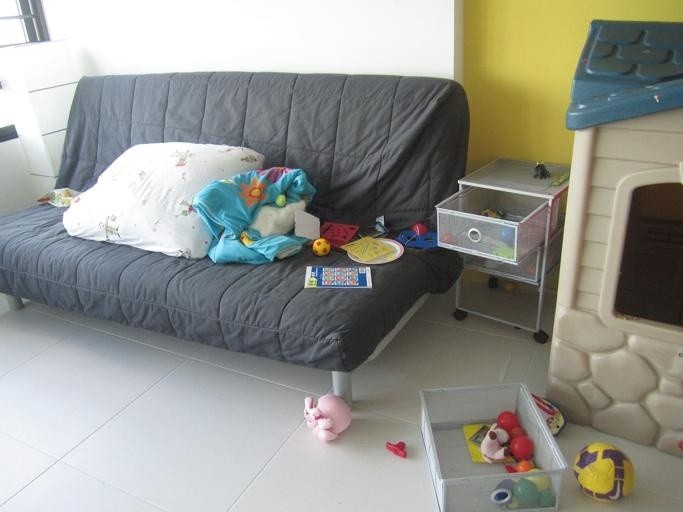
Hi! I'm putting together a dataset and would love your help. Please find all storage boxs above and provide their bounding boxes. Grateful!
[420,381,568,511]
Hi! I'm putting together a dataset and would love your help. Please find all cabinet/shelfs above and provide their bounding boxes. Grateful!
[432,155,569,343]
[0,39,97,200]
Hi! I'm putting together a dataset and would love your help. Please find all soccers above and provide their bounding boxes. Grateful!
[574,442,636,503]
[313,239,330,257]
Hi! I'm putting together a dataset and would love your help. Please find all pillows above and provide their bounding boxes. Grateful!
[62,140,266,264]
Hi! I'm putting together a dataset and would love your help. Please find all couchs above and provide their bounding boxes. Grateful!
[0,71,470,413]
